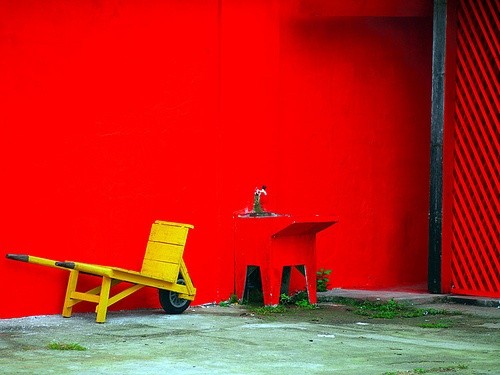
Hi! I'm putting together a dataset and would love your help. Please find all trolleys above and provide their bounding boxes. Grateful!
[7,220,197,322]
